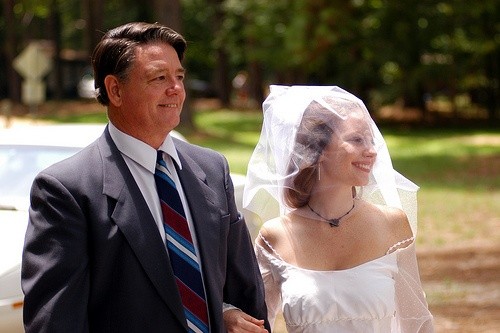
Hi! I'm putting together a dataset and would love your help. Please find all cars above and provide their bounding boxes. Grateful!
[0,123,187,333]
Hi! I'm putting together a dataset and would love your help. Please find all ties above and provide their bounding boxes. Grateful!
[155,150,210,332]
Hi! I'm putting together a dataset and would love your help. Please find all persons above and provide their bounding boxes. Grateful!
[219,85,434,333]
[13,19,270,333]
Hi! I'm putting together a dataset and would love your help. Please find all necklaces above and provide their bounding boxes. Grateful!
[308,202,355,229]
